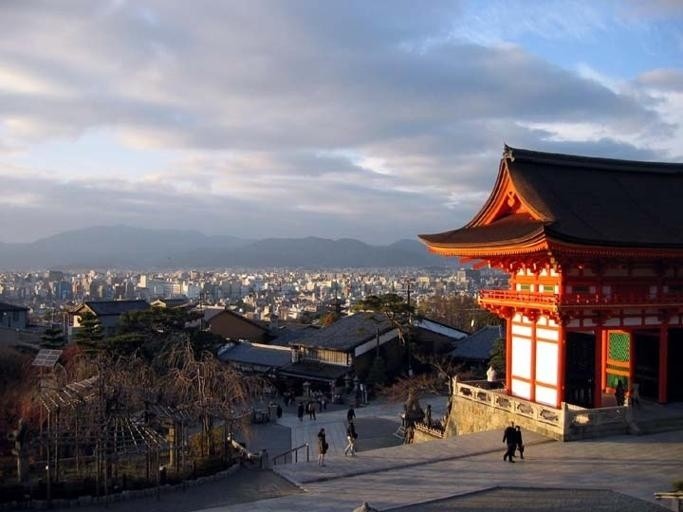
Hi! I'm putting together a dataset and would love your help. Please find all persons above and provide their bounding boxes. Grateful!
[347,405,356,423]
[276,389,329,422]
[158,464,164,473]
[344,419,358,457]
[614,379,625,406]
[486,362,498,388]
[316,428,328,467]
[513,426,524,459]
[160,467,166,486]
[424,405,431,429]
[502,421,517,463]
[629,384,642,408]
